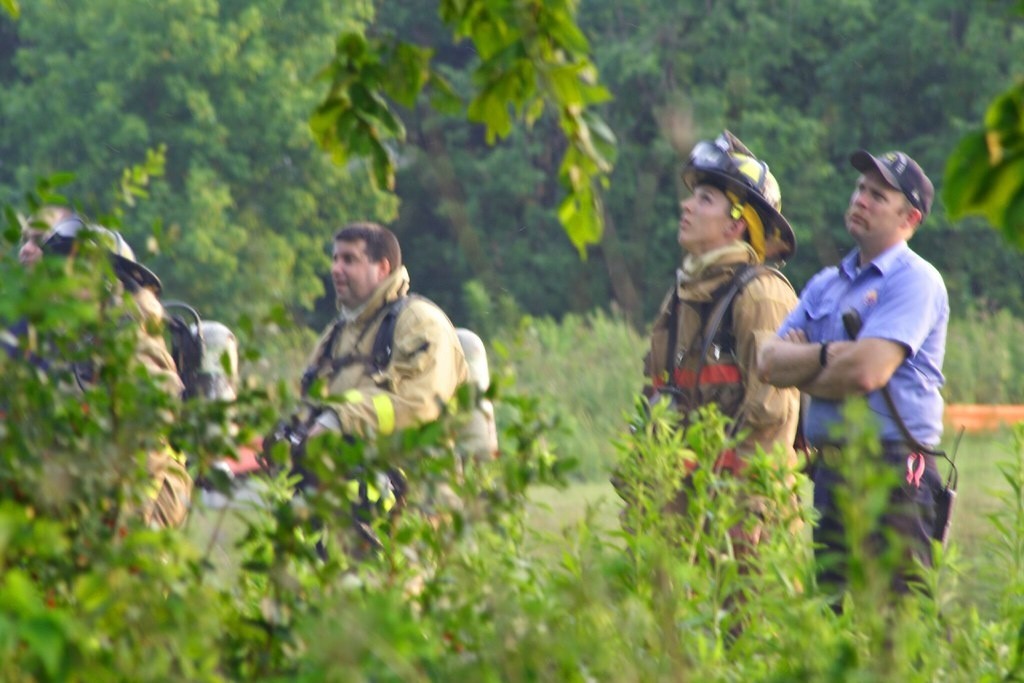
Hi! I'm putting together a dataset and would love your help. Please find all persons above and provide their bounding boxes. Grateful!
[0,207,193,582]
[755,151,949,616]
[610,154,802,645]
[295,222,466,611]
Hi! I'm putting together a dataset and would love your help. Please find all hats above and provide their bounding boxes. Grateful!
[684,135,795,259]
[850,148,937,216]
[39,221,165,294]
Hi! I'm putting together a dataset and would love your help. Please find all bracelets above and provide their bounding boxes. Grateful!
[820,340,834,370]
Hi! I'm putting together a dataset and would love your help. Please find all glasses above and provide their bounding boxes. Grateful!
[37,216,86,247]
[688,140,767,202]
